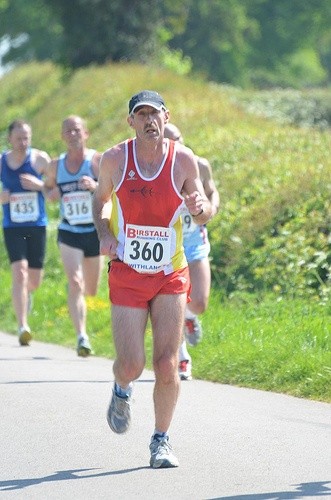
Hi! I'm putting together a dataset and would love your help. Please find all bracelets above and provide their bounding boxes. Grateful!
[192,210,203,217]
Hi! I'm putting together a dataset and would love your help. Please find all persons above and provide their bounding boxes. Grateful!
[0,120,51,346]
[164,123,220,380]
[91,90,212,465]
[43,115,113,357]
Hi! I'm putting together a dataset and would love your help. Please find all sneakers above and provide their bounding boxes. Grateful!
[106,380,133,433]
[76,337,91,357]
[183,317,203,346]
[18,328,32,346]
[149,435,179,469]
[27,294,32,312]
[178,357,192,380]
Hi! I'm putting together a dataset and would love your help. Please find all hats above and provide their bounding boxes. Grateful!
[128,90,167,116]
